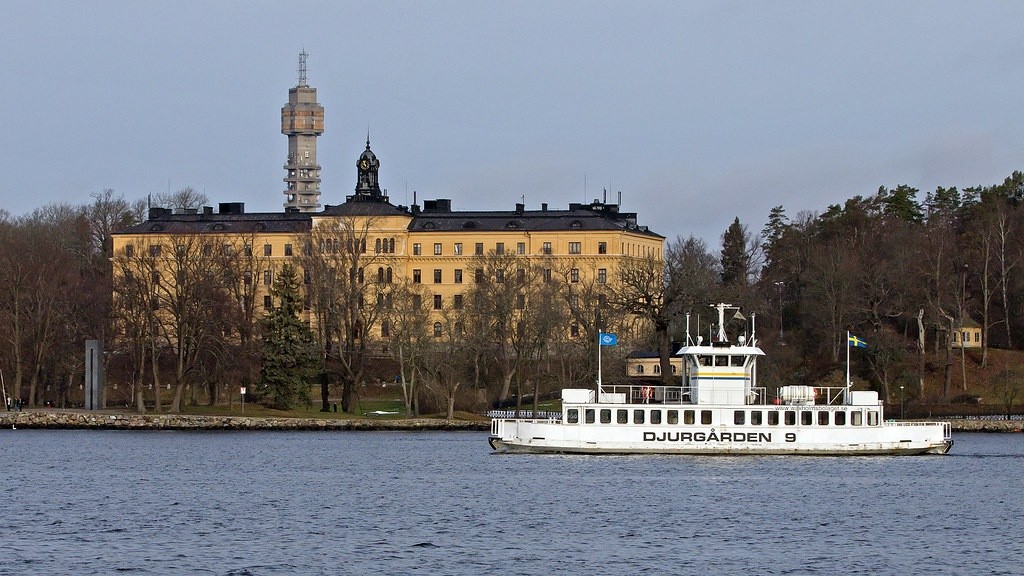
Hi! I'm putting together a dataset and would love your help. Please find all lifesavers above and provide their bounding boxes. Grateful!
[814,389,818,398]
[643,387,652,398]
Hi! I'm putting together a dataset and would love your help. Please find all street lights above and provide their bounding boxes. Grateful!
[775,282,785,336]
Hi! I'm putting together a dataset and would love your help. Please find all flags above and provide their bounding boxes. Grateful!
[849,334,868,349]
[600,334,617,346]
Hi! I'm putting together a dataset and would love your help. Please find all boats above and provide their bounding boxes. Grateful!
[485,302,956,457]
